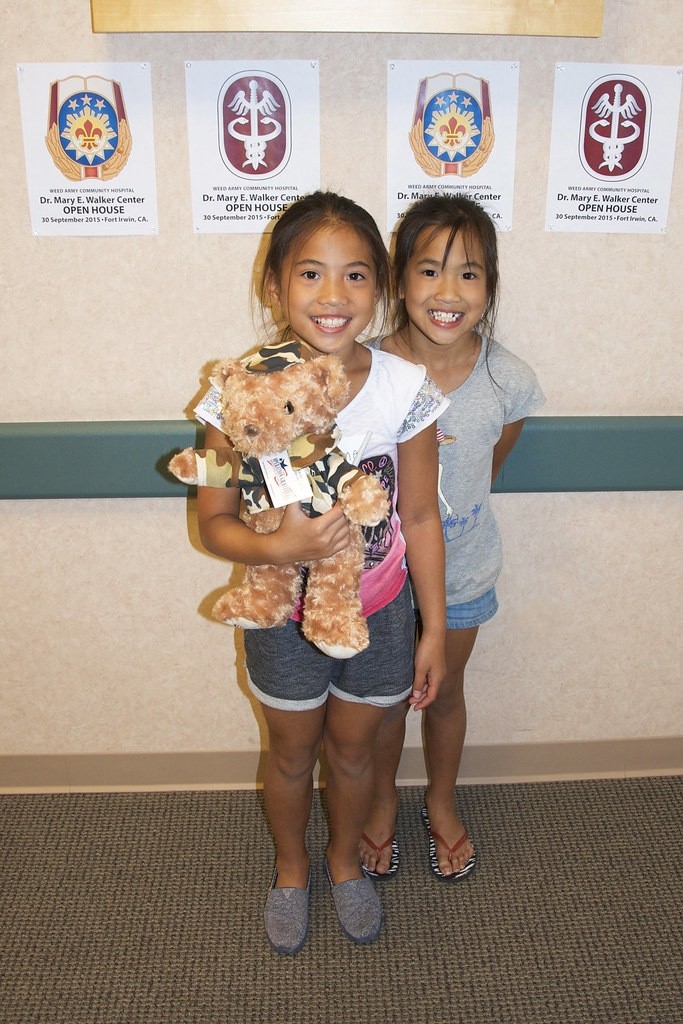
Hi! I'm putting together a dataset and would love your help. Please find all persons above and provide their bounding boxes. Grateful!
[193,192,452,951]
[358,197,547,882]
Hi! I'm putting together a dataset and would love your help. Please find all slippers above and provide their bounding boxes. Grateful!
[358,797,399,878]
[419,805,477,880]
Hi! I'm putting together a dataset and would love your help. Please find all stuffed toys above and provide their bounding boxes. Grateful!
[168,339,390,661]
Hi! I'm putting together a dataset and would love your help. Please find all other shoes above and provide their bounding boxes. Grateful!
[324,855,383,942]
[264,861,314,951]
